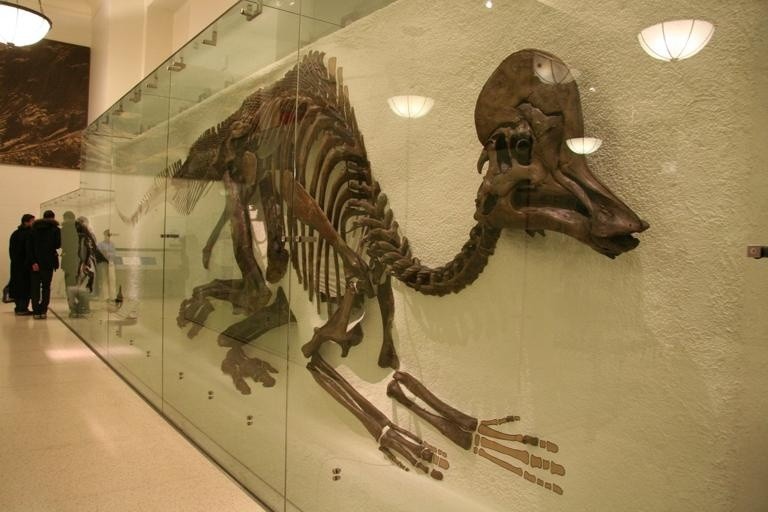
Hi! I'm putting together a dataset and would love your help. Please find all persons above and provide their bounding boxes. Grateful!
[8,214,41,316]
[60,211,91,319]
[26,210,62,320]
[75,214,94,293]
[96,229,115,249]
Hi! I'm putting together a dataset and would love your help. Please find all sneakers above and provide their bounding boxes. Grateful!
[40,313,47,320]
[33,314,40,318]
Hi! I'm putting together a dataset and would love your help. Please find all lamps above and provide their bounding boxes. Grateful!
[0,0,50,49]
[637,18,715,64]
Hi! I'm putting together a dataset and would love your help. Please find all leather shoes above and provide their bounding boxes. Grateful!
[16,310,33,316]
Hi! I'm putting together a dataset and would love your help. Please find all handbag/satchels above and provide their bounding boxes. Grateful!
[2,286,16,303]
